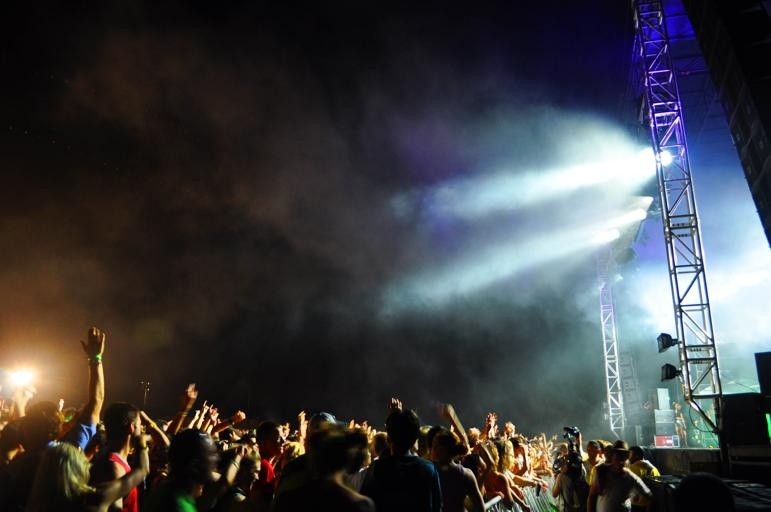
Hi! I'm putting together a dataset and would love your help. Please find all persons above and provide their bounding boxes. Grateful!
[1,381,733,512]
[25,326,107,451]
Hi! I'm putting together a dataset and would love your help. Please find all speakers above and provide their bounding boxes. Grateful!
[718,393,771,448]
[755,351,771,393]
[653,388,681,449]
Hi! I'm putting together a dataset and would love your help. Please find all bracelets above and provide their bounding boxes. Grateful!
[87,352,103,365]
